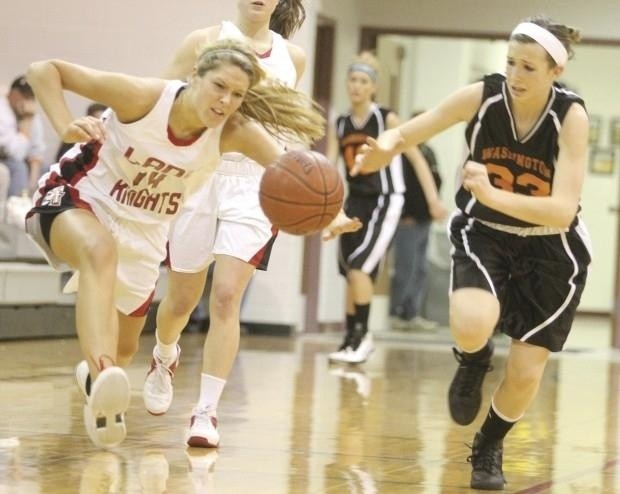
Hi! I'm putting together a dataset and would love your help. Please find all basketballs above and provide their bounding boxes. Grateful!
[259,150,344,237]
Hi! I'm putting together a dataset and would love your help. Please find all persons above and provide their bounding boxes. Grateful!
[55,102,107,162]
[327,51,448,364]
[0,74,46,225]
[349,14,594,492]
[20,38,365,448]
[143,0,313,447]
[388,105,449,330]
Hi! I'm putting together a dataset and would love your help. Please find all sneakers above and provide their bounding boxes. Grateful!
[74,353,130,449]
[143,344,181,416]
[448,348,494,425]
[464,432,506,490]
[329,331,372,364]
[186,404,219,448]
